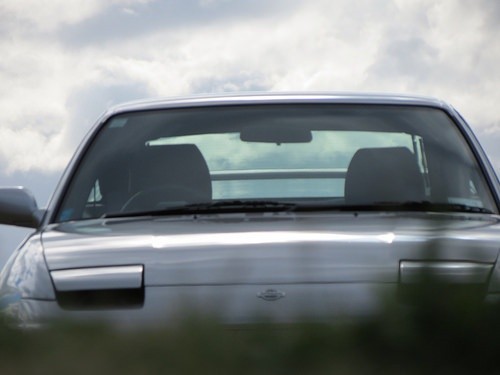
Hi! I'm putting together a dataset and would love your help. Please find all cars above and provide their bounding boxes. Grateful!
[0,89,500,347]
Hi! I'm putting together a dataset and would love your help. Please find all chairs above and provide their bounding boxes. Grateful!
[127,144,212,211]
[343,147,426,204]
